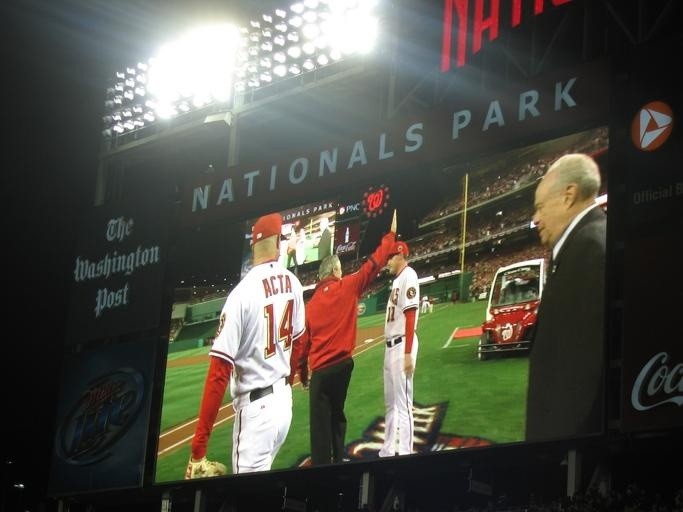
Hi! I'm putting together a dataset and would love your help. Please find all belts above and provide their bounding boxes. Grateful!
[383,337,406,348]
[233,375,292,404]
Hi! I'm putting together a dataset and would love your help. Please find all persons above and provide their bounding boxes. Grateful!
[421,293,429,313]
[361,241,420,458]
[287,216,306,267]
[186,213,307,477]
[408,155,552,300]
[287,207,397,467]
[312,216,331,260]
[524,152,608,441]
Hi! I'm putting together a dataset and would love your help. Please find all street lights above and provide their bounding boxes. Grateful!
[93,1,402,205]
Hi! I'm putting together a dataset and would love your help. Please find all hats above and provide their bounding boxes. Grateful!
[386,240,409,258]
[249,212,290,245]
